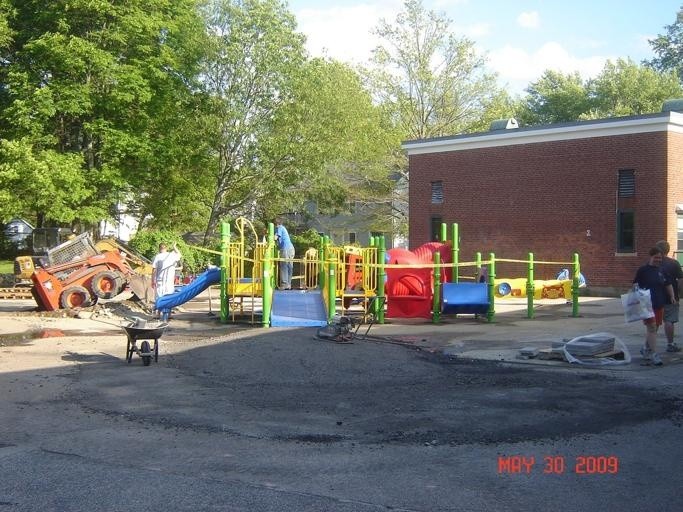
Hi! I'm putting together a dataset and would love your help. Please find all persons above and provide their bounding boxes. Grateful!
[151,241,182,319]
[640,241,683,354]
[571,272,587,291]
[557,269,569,280]
[272,218,295,290]
[632,247,676,366]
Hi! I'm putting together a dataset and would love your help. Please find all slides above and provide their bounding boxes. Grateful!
[153,268,223,310]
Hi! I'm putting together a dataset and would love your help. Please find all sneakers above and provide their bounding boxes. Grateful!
[639,342,682,366]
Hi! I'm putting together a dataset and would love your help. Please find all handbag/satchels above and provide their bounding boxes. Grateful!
[620,287,656,323]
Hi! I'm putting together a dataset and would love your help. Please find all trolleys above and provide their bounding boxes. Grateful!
[119,316,169,365]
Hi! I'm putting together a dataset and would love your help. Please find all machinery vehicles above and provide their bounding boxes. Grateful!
[14,228,184,312]
